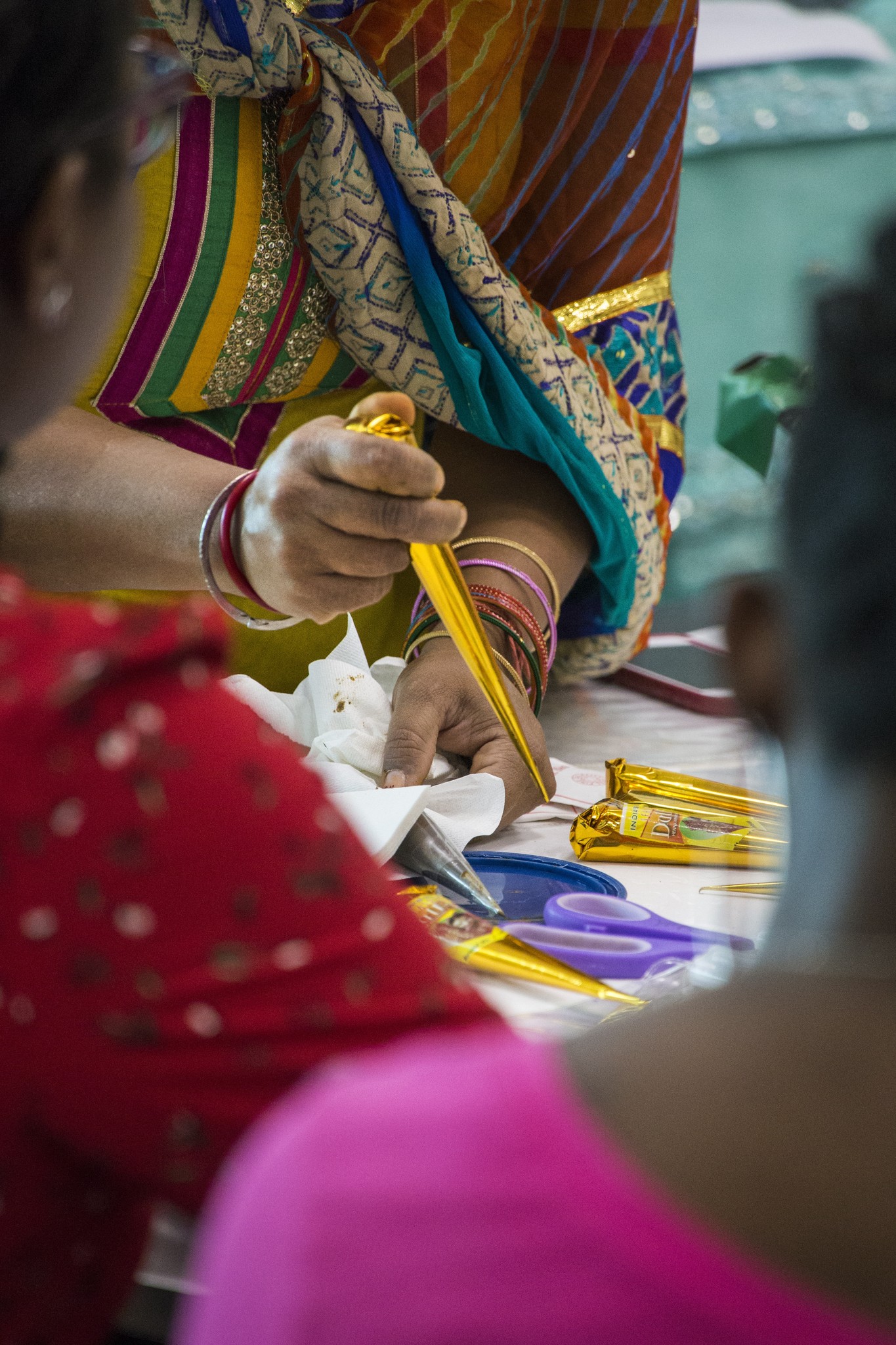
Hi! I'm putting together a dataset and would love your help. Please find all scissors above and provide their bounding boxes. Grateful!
[500,890,755,980]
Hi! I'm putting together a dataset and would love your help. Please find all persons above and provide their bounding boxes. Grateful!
[180,200,896,1345]
[0,22,505,1345]
[0,0,691,830]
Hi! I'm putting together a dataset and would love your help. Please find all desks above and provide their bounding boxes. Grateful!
[143,624,804,1284]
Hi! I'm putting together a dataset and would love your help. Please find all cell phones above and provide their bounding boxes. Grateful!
[610,633,743,718]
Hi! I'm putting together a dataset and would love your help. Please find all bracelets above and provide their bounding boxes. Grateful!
[411,559,558,691]
[219,470,277,612]
[198,470,306,631]
[449,535,561,644]
[399,585,549,716]
